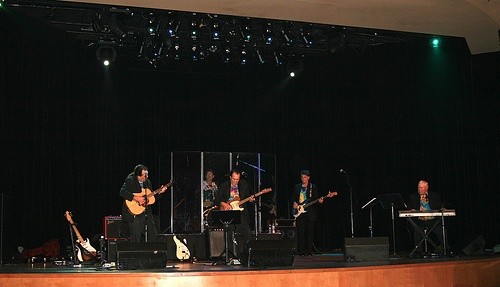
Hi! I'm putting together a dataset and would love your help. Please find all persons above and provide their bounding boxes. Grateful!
[118,164,168,242]
[291,169,324,257]
[200,168,218,219]
[406,179,455,258]
[217,169,256,266]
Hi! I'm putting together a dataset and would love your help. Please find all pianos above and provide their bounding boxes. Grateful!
[398,209,456,259]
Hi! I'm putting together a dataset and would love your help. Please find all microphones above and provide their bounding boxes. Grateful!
[340,169,346,174]
[242,172,247,175]
[236,154,239,166]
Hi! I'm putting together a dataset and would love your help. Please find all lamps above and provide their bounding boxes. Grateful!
[96,46,117,67]
[286,59,305,78]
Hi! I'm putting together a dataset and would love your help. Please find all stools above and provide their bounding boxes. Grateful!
[24,238,60,263]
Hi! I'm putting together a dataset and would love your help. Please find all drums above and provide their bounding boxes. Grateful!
[202,188,219,230]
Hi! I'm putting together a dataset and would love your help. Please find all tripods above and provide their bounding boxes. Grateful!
[211,209,241,266]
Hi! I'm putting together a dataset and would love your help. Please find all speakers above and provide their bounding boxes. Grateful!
[103,216,129,240]
[116,241,167,271]
[462,235,486,256]
[205,227,236,258]
[343,237,389,262]
[239,239,295,267]
[275,226,299,255]
[108,241,116,262]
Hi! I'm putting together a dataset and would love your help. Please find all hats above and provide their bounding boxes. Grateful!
[300,170,310,176]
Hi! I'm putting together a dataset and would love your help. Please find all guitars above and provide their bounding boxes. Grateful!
[292,191,337,217]
[64,210,97,262]
[172,213,191,260]
[219,188,272,212]
[124,179,174,217]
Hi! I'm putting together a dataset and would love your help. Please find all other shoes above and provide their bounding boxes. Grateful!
[299,254,305,256]
[307,253,312,256]
